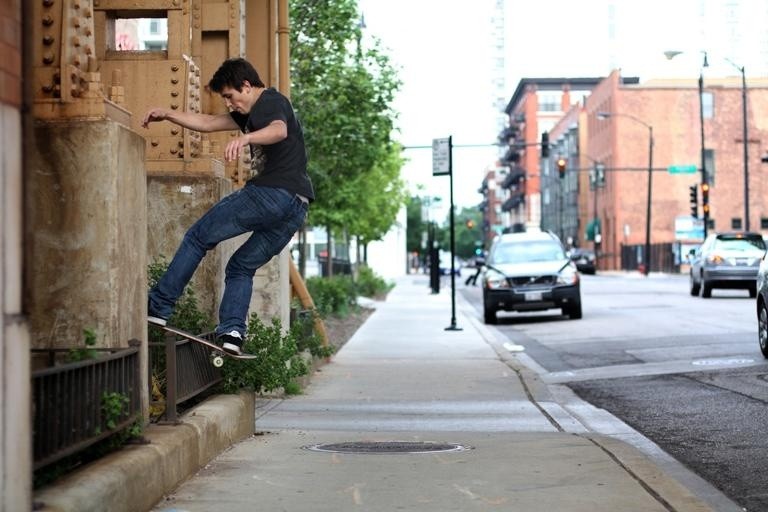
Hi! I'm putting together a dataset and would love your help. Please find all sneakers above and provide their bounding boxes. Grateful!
[222,329,244,356]
[148,312,172,327]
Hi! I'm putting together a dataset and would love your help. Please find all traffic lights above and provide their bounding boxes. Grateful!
[690,184,709,219]
[466,220,473,229]
[558,158,567,179]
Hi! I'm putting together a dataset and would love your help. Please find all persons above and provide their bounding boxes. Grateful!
[141,58,315,367]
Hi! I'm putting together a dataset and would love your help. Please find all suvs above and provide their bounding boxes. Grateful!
[477,228,582,323]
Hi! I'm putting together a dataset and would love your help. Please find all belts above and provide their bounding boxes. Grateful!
[286,188,309,211]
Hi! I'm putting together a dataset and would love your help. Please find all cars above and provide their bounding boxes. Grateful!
[439,251,460,276]
[688,232,767,298]
[571,248,596,274]
[756,253,768,358]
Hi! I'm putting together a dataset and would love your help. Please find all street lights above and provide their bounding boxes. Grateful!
[666,49,751,230]
[597,112,654,273]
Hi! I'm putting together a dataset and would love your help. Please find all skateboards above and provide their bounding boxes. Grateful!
[148,320,258,368]
